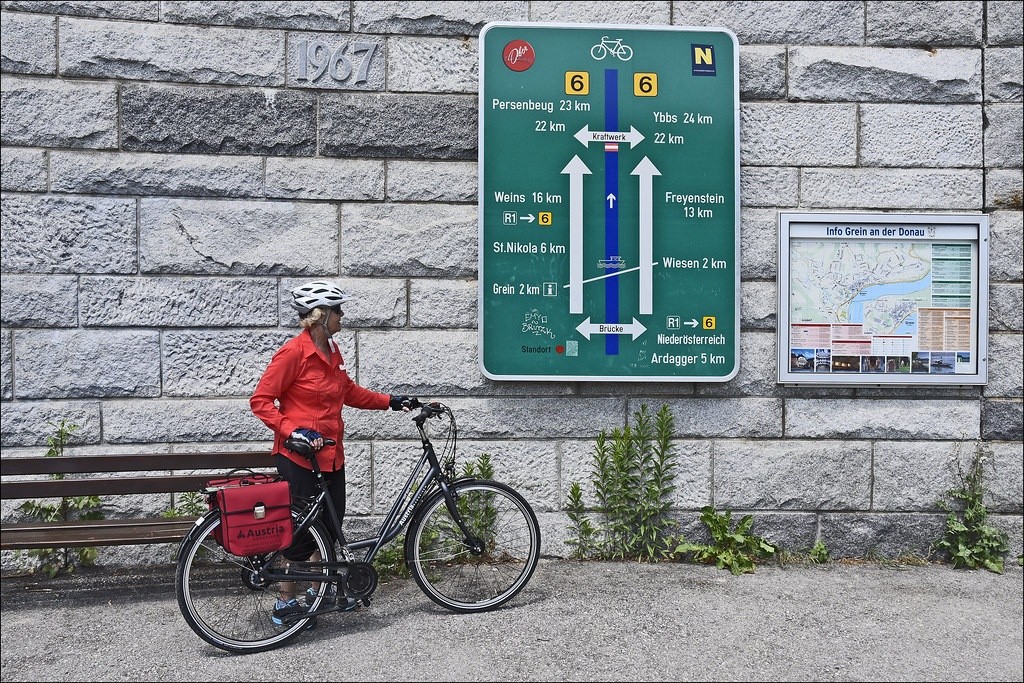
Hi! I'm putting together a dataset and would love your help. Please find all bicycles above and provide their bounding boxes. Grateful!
[173,398,543,652]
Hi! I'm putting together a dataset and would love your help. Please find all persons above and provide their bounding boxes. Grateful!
[249,281,410,629]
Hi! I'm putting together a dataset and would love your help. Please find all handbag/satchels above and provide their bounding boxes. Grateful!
[207,468,293,557]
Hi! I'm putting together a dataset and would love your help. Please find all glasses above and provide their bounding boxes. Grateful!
[317,305,342,314]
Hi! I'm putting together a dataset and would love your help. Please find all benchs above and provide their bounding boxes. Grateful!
[0,451,278,549]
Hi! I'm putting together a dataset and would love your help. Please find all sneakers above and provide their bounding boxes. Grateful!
[271,597,317,628]
[305,583,356,611]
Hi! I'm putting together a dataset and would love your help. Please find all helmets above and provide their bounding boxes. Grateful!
[289,281,347,314]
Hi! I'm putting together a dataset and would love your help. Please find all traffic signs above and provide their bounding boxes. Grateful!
[477,21,742,384]
